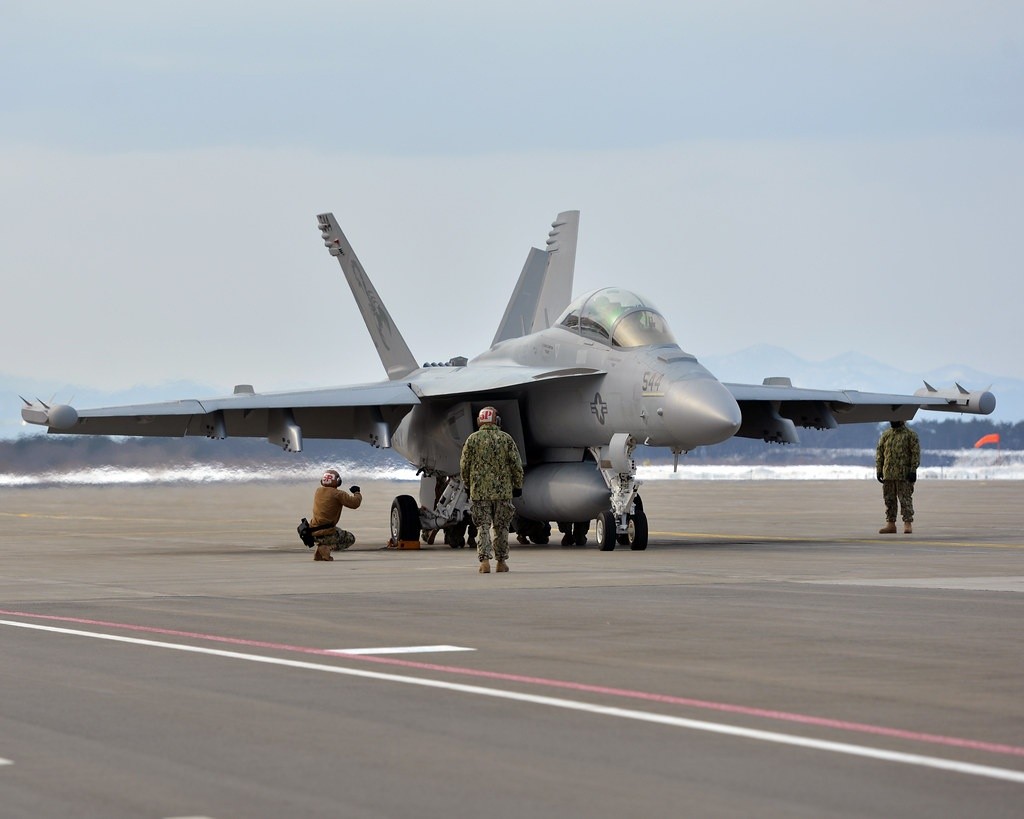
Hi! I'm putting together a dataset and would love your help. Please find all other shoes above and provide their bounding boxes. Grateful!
[467,537,477,548]
[516,535,530,545]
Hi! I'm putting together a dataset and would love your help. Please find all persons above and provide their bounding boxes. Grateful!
[460,406,524,573]
[309,469,363,561]
[875,421,921,534]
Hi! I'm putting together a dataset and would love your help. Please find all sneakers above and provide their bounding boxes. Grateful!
[880,521,897,533]
[318,544,333,560]
[314,550,323,560]
[496,560,509,572]
[478,560,489,573]
[903,521,912,533]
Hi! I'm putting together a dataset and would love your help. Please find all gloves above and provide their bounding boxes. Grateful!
[908,472,916,483]
[511,487,522,498]
[349,485,360,493]
[465,488,470,499]
[877,472,884,483]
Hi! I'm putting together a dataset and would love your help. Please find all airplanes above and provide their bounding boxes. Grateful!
[21,211,997,552]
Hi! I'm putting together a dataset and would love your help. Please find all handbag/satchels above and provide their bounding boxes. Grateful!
[297,517,314,548]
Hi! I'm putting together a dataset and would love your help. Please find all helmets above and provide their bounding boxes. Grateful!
[477,406,501,426]
[320,469,342,487]
